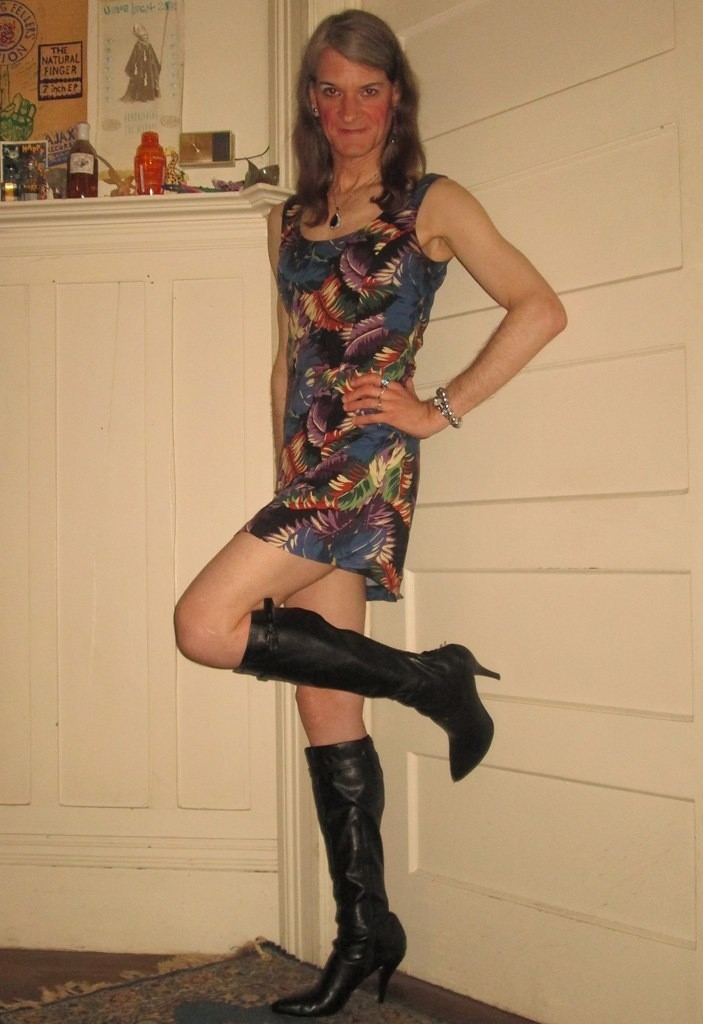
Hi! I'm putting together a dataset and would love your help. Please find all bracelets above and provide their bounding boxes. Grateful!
[434,388,462,428]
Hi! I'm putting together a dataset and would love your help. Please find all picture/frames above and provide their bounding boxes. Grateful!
[0,0,103,171]
[0,140,48,183]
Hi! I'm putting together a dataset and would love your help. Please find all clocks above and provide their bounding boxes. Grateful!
[178,130,236,169]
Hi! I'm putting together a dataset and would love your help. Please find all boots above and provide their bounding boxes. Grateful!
[272,736,408,1024]
[235,596,501,783]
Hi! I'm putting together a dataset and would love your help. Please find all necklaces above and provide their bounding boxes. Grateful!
[328,170,382,228]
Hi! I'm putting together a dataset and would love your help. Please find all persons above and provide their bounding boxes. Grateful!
[173,9,569,1019]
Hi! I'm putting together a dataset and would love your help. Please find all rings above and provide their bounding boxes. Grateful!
[378,389,386,398]
[381,374,392,387]
[376,399,382,410]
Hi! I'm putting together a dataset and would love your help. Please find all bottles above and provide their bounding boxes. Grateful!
[134,132,166,195]
[18,163,37,201]
[67,123,98,197]
[5,167,18,202]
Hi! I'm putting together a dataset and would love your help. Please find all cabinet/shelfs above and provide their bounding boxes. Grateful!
[0,182,301,956]
